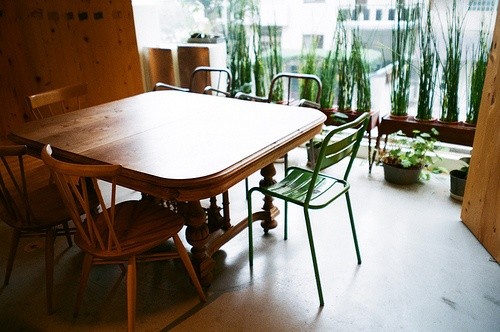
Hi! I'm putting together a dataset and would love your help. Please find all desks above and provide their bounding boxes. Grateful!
[7,90,328,290]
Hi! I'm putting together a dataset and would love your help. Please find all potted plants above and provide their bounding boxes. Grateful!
[306,112,348,167]
[216,0,492,146]
[377,127,441,186]
[449,149,473,201]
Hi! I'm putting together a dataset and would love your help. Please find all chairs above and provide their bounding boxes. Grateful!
[248,112,371,306]
[234,73,322,200]
[153,67,233,97]
[0,83,206,332]
